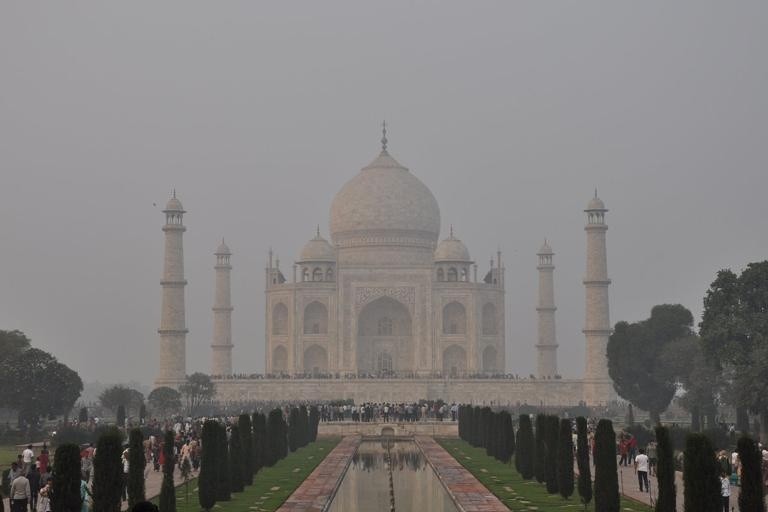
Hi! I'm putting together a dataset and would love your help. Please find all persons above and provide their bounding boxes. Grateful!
[351,452,428,473]
[677,450,686,479]
[570,416,657,492]
[716,422,767,512]
[317,402,457,424]
[0,403,319,512]
